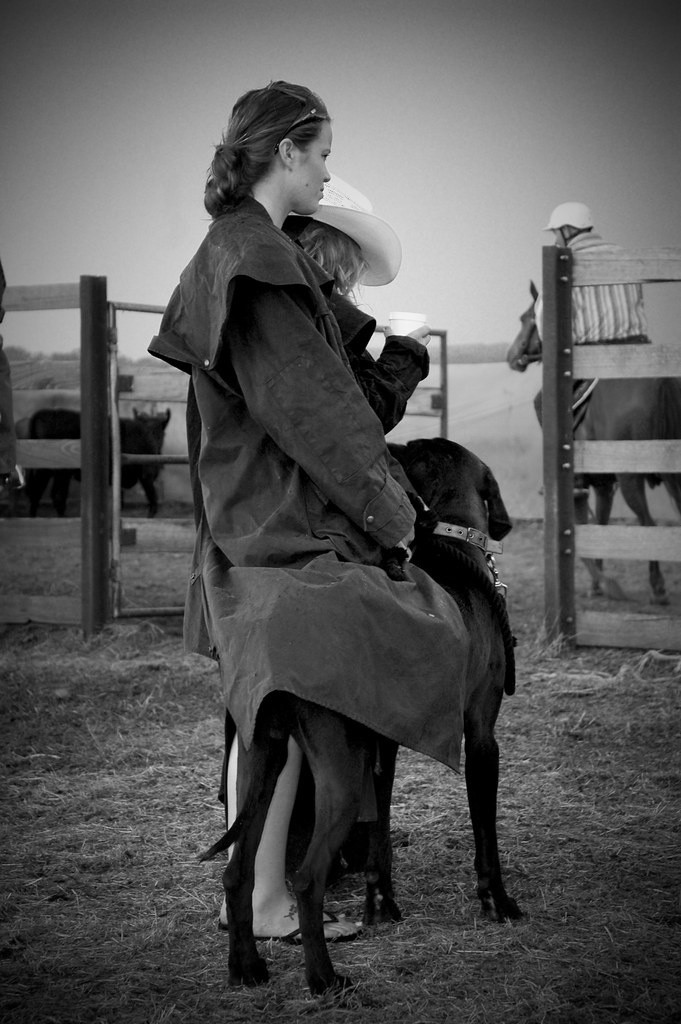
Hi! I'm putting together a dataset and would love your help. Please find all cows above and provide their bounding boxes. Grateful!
[26,406,172,517]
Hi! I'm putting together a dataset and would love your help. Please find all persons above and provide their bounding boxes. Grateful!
[150,81,469,941]
[532,201,648,428]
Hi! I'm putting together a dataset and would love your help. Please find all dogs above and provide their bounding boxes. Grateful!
[196,436,516,997]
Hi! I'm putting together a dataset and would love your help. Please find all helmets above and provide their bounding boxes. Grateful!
[544,202,594,230]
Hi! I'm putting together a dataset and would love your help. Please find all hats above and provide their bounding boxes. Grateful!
[289,171,402,286]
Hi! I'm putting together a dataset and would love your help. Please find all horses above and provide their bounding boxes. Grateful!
[507,280,681,604]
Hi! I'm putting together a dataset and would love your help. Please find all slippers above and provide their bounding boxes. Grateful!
[217,919,230,931]
[252,911,361,942]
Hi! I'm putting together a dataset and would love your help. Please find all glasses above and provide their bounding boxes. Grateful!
[274,92,329,153]
[358,255,370,280]
